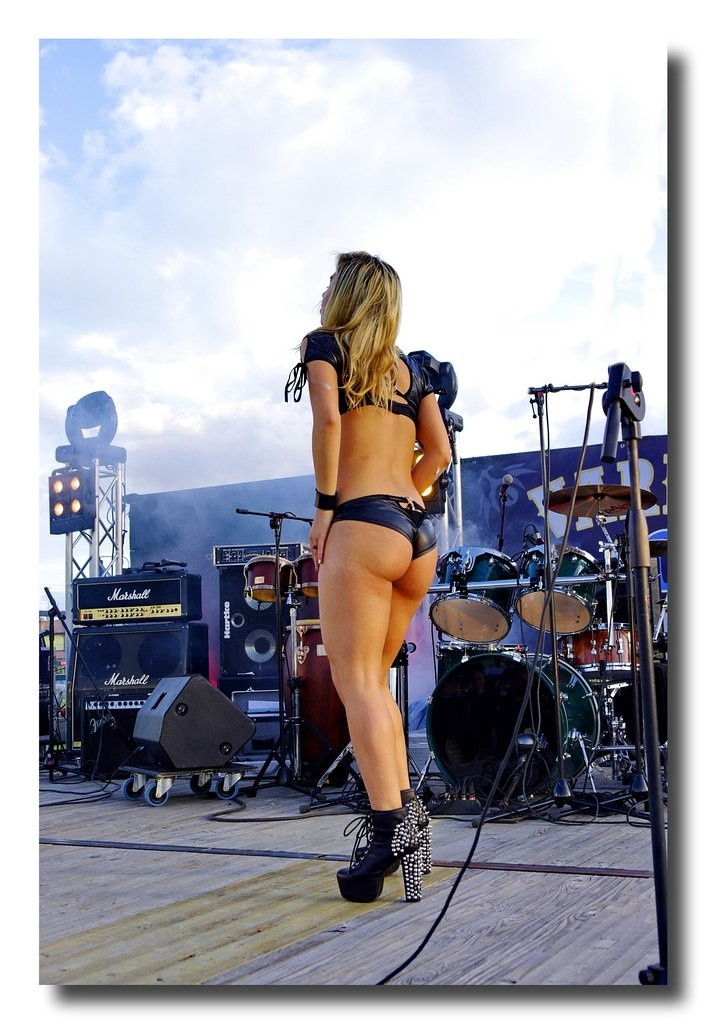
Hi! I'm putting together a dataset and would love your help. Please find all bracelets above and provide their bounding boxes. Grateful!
[314,488,338,510]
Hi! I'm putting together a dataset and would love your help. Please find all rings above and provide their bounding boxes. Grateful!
[312,544,318,549]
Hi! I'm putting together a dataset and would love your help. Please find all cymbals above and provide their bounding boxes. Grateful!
[542,483,658,519]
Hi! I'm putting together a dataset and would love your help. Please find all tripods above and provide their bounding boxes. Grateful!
[236,507,434,803]
[468,381,652,831]
[39,587,116,784]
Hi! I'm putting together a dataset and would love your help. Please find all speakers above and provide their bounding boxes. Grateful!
[81,694,158,780]
[133,675,257,773]
[219,566,290,676]
[67,622,208,756]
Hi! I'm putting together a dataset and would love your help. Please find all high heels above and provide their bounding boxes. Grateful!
[356,786,432,873]
[338,807,422,902]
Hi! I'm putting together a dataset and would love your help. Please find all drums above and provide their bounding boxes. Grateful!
[292,552,320,598]
[435,643,529,685]
[515,542,604,636]
[423,650,602,804]
[555,621,643,687]
[283,618,353,788]
[244,555,296,603]
[428,547,521,644]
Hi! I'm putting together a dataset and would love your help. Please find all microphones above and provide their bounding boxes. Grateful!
[109,720,130,741]
[499,474,512,499]
[533,526,545,546]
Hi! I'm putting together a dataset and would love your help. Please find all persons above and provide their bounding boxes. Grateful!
[284,252,452,903]
